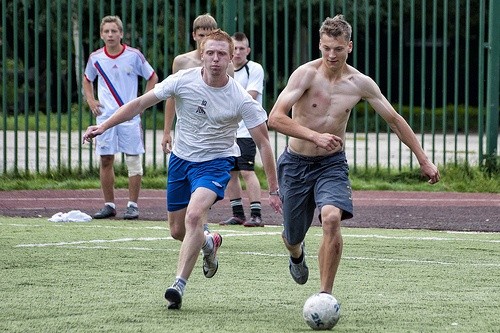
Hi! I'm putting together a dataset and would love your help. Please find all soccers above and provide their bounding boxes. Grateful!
[303,293,340,330]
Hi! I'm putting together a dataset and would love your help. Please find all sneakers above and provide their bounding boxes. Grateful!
[165,276,187,310]
[243,215,265,227]
[219,215,245,225]
[289,239,309,285]
[93,204,117,218]
[122,204,139,220]
[203,233,222,278]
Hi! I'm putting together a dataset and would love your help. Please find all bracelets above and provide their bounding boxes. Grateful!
[270,191,279,196]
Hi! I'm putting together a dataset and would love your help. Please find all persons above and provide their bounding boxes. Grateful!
[82,14,159,220]
[161,14,234,154]
[82,29,283,310]
[219,32,264,227]
[267,14,441,295]
